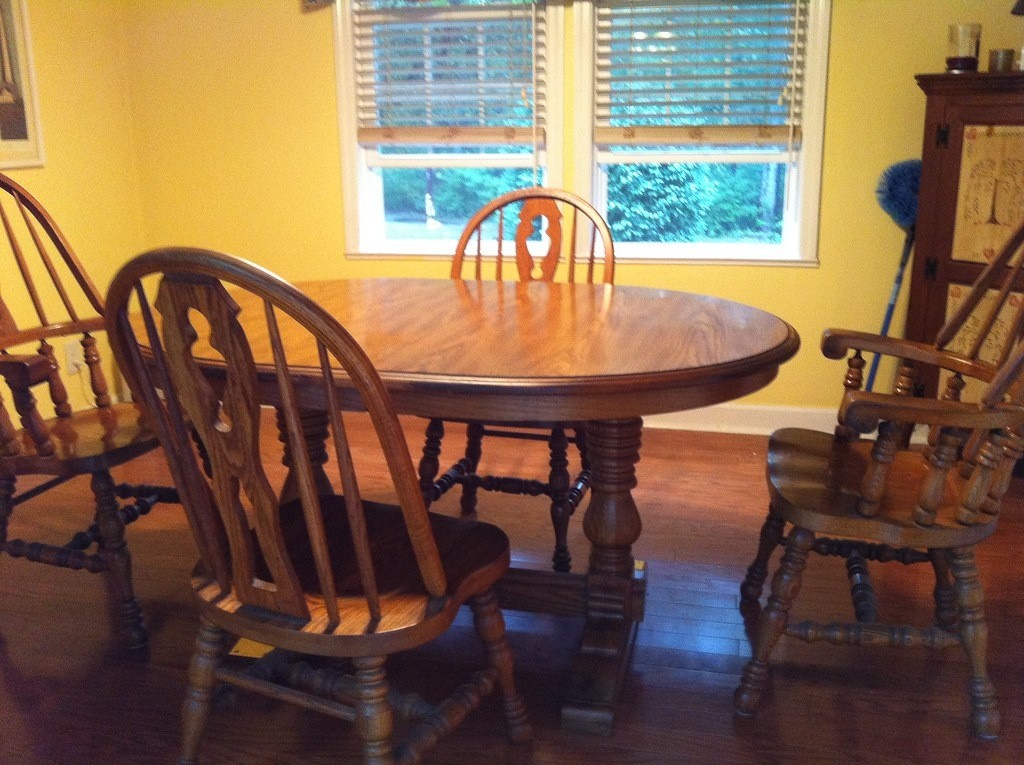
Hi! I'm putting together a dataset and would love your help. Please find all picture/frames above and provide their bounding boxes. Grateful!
[0,0,47,168]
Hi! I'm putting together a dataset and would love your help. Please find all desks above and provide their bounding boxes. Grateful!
[121,279,801,736]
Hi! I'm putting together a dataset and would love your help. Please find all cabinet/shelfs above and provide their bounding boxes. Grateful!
[902,71,1024,452]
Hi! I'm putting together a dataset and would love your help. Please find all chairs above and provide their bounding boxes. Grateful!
[0,173,232,674]
[735,228,1024,745]
[415,187,624,571]
[106,249,536,764]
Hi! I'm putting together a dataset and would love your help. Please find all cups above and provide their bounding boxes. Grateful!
[945,23,982,74]
[988,48,1013,74]
